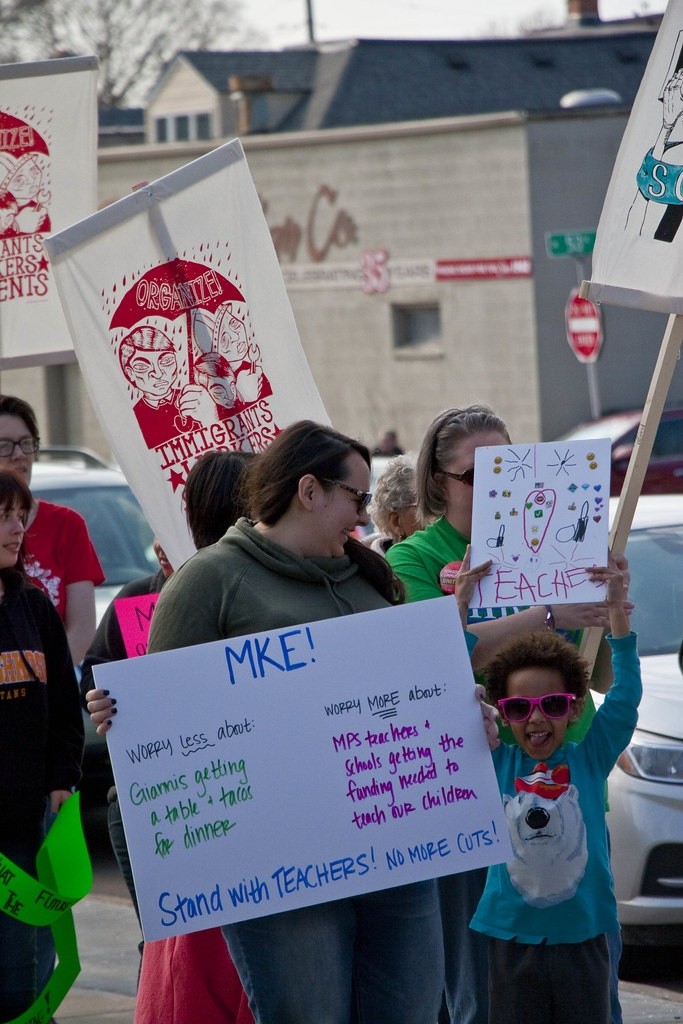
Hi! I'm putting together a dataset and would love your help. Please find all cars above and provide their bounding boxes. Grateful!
[346,454,419,542]
[543,492,683,947]
[555,407,683,496]
[29,446,158,863]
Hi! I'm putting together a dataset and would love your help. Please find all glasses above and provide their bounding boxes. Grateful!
[0,437,40,457]
[435,468,474,487]
[498,693,576,723]
[318,476,373,515]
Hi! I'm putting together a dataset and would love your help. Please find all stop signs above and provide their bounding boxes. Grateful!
[565,289,603,364]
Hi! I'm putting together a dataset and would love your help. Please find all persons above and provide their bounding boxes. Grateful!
[78,450,263,1024]
[454,544,643,1024]
[383,403,633,1024]
[359,453,424,559]
[372,431,403,456]
[85,420,500,1024]
[0,393,106,1024]
[0,469,85,1024]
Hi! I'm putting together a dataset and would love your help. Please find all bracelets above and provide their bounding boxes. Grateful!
[544,604,555,631]
